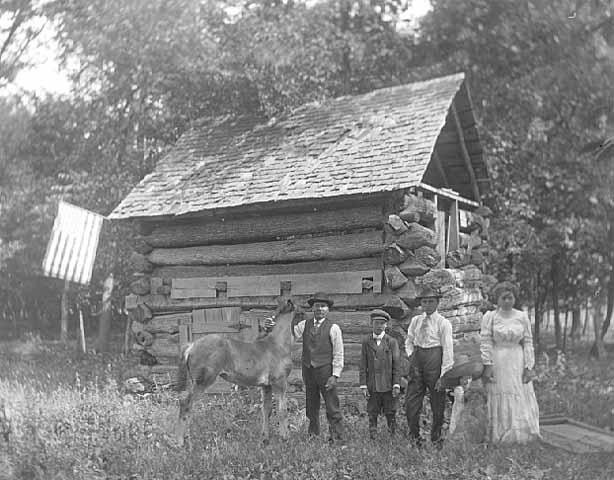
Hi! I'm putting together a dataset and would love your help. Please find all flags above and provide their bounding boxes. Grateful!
[39,200,102,288]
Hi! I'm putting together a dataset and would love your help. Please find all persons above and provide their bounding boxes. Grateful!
[357,308,401,440]
[478,282,543,446]
[265,291,345,441]
[441,353,484,402]
[403,287,454,446]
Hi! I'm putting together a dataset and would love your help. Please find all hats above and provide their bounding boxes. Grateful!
[307,292,334,307]
[416,288,442,299]
[440,355,484,387]
[369,309,390,323]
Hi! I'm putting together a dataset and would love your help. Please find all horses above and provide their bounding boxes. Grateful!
[176,296,310,447]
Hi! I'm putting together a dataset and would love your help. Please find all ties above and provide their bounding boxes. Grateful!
[314,321,320,332]
